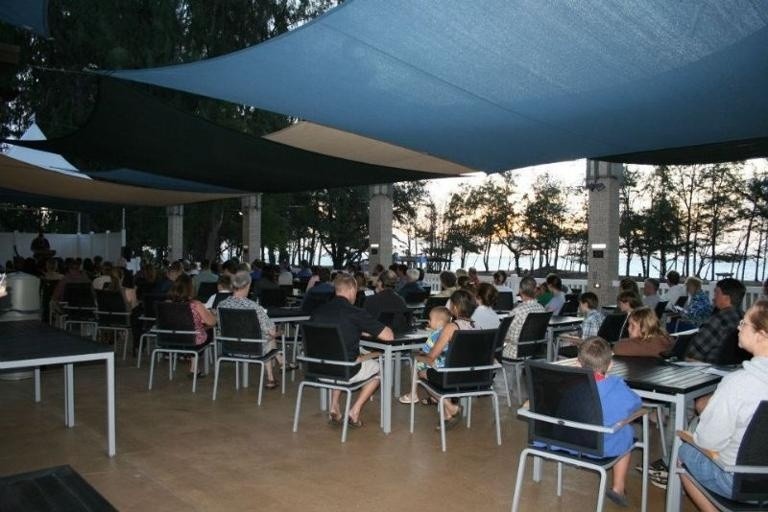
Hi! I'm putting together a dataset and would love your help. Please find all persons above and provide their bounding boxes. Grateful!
[163,257,217,273]
[162,274,217,378]
[642,277,674,312]
[545,273,565,317]
[470,282,501,329]
[217,270,298,390]
[494,276,546,396]
[435,270,456,297]
[206,275,233,309]
[396,306,452,405]
[457,274,476,293]
[596,277,645,341]
[616,290,645,339]
[612,307,675,357]
[456,269,466,278]
[468,267,479,283]
[222,260,237,276]
[522,335,641,507]
[310,273,394,428]
[251,253,297,281]
[683,277,754,364]
[294,260,312,277]
[663,270,686,305]
[398,268,426,303]
[301,268,334,310]
[557,292,605,359]
[353,258,404,286]
[492,270,516,302]
[363,270,409,332]
[1,232,163,358]
[681,276,714,321]
[676,300,768,512]
[414,289,476,433]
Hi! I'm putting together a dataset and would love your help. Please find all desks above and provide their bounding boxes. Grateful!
[266,308,310,381]
[1,318,116,458]
[360,325,435,437]
[549,337,744,512]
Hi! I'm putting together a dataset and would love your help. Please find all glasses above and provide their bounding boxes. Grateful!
[739,319,766,339]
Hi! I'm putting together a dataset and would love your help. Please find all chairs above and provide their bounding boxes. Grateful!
[64,282,97,337]
[492,315,515,407]
[511,362,652,512]
[194,282,219,303]
[555,336,668,457]
[559,303,579,316]
[675,401,768,512]
[280,286,292,294]
[672,339,691,361]
[654,300,669,319]
[212,306,284,406]
[297,276,311,291]
[494,292,513,313]
[423,287,431,295]
[94,288,133,361]
[257,287,286,308]
[410,327,502,452]
[293,322,384,443]
[148,301,209,393]
[675,296,689,307]
[551,312,631,362]
[353,290,377,309]
[496,311,553,406]
[377,309,412,331]
[137,292,169,369]
[407,296,452,328]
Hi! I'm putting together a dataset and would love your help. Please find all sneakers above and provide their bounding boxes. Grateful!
[279,362,298,373]
[399,394,419,404]
[453,404,463,418]
[636,461,665,477]
[422,397,437,406]
[606,488,629,507]
[265,379,280,389]
[187,370,206,379]
[341,413,368,431]
[326,413,344,427]
[650,472,684,495]
[435,417,456,433]
